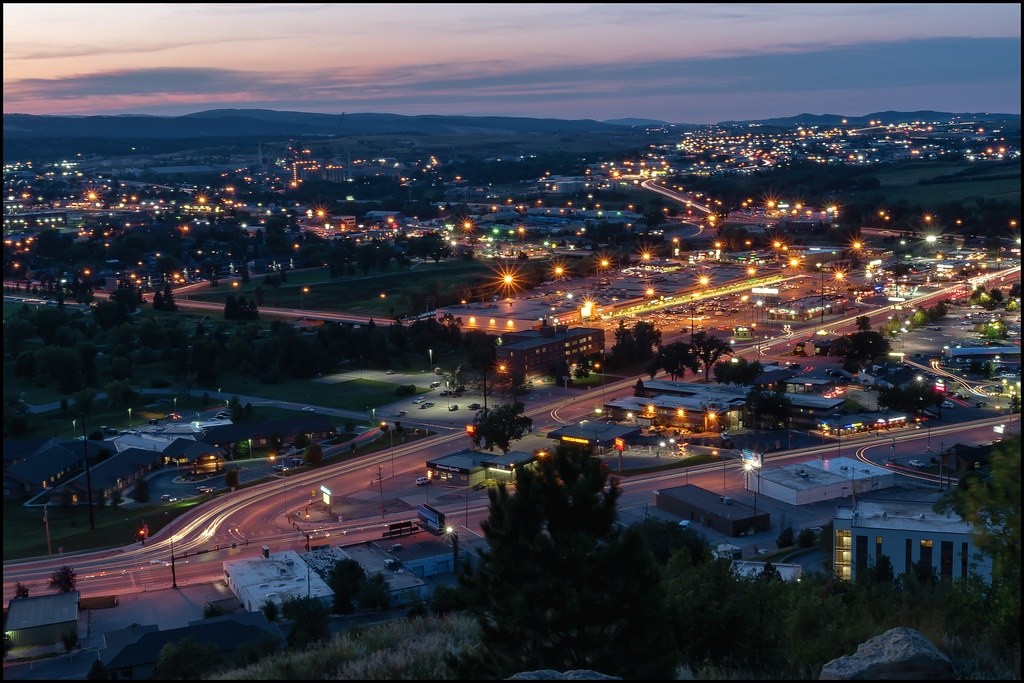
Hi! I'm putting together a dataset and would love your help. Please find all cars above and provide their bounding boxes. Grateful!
[414,397,426,404]
[160,495,177,503]
[386,369,396,375]
[439,386,465,398]
[273,465,289,472]
[430,382,440,388]
[166,413,183,422]
[395,410,408,418]
[416,477,432,487]
[302,406,316,413]
[448,405,458,411]
[216,411,233,420]
[599,294,748,332]
[468,403,481,410]
[196,486,213,495]
[421,402,435,409]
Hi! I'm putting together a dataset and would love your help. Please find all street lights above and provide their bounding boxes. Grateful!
[896,235,905,296]
[820,266,844,324]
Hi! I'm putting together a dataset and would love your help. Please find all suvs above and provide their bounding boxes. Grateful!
[909,459,926,469]
[291,458,305,467]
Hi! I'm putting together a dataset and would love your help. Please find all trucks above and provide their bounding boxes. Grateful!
[810,527,824,535]
[678,520,691,531]
